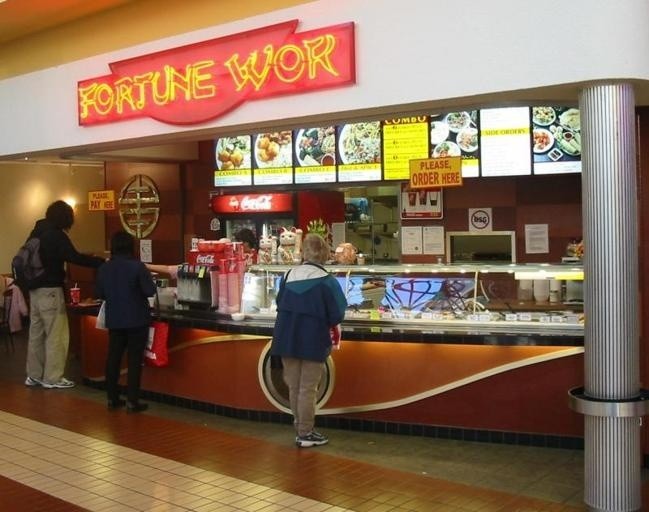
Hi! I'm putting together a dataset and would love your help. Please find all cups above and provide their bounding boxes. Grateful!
[148,296,155,311]
[69,288,80,304]
[320,154,335,166]
[219,242,245,310]
[408,191,438,206]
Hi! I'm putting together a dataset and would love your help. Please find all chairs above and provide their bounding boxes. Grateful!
[0,278,17,354]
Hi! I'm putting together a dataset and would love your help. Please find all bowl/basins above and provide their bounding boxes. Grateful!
[519,279,562,303]
[231,313,245,321]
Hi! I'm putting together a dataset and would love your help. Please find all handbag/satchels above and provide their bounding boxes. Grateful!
[95,300,107,330]
[143,320,169,369]
[330,322,342,351]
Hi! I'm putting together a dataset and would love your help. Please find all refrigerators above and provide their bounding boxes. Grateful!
[211,191,345,253]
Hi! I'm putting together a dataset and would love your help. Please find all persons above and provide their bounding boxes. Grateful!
[235,228,258,264]
[269,233,347,448]
[89,229,157,415]
[23,200,105,389]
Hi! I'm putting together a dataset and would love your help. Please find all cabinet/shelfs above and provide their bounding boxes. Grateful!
[239,263,586,337]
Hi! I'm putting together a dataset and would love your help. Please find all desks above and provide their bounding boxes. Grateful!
[65,301,101,351]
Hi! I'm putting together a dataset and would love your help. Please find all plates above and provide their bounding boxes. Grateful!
[533,107,581,156]
[431,112,478,157]
[294,128,335,166]
[214,135,251,170]
[338,121,381,164]
[254,133,292,168]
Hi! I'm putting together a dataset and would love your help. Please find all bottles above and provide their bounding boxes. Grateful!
[356,252,366,265]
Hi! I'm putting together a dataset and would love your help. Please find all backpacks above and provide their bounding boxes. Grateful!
[11,237,46,291]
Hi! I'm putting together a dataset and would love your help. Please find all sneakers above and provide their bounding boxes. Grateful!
[42,376,75,388]
[296,432,329,444]
[299,431,329,447]
[24,376,42,386]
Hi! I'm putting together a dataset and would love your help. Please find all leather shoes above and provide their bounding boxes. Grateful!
[108,400,126,409]
[126,402,148,412]
